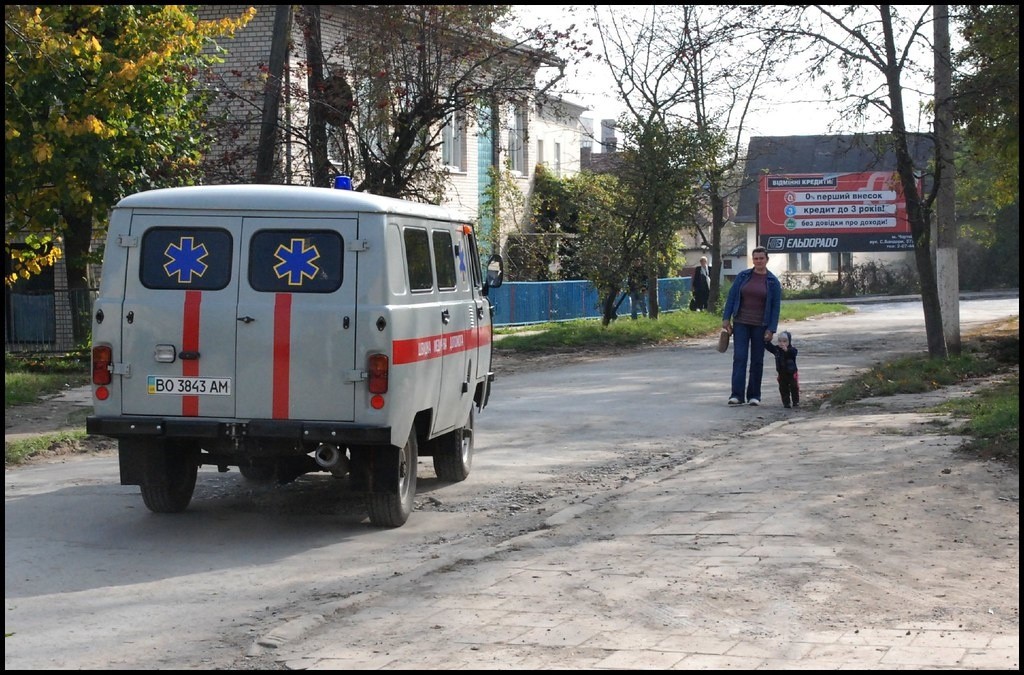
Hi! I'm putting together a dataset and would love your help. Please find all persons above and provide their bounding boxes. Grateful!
[691,256,712,311]
[765,331,799,408]
[722,247,781,405]
[597,256,650,320]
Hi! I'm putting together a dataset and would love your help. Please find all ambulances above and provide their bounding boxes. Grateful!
[85,173,504,529]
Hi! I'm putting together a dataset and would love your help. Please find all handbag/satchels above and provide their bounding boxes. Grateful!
[689,300,697,311]
[716,328,730,353]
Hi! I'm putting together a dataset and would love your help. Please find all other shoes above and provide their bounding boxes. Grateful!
[794,403,799,406]
[727,398,743,404]
[750,399,760,406]
[785,404,791,408]
